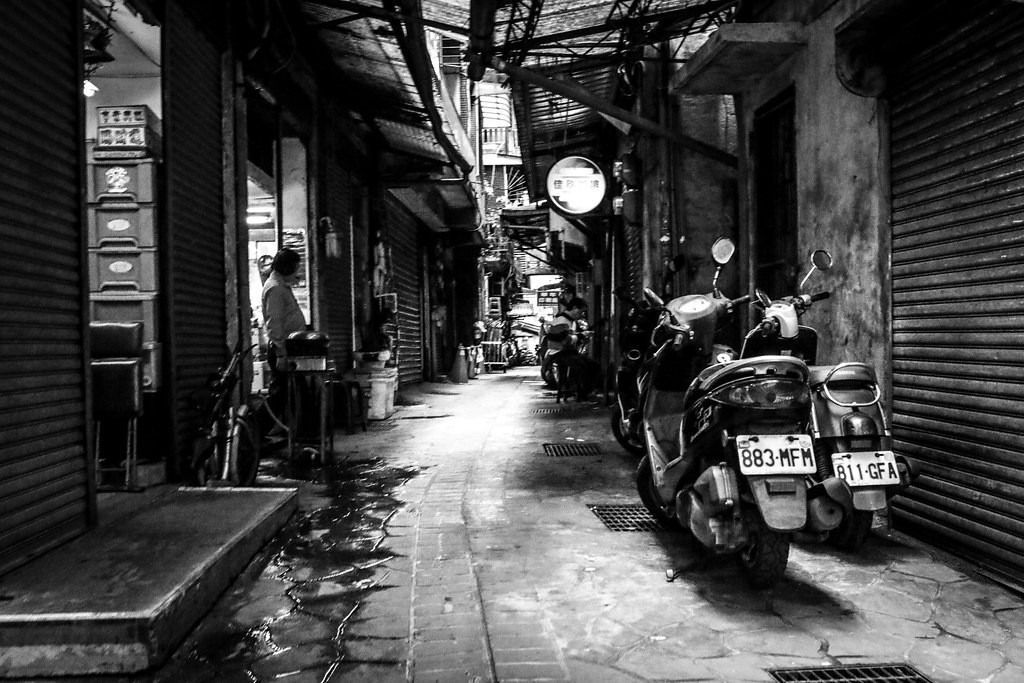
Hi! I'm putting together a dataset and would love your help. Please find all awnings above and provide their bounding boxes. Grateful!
[293,0,475,189]
[459,1,740,249]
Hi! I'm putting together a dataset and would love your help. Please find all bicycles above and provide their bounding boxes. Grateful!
[188,337,263,487]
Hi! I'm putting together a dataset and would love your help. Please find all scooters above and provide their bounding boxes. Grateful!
[609,237,816,590]
[740,249,903,552]
[538,317,594,390]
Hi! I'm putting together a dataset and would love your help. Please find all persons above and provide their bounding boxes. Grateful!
[252,248,308,440]
